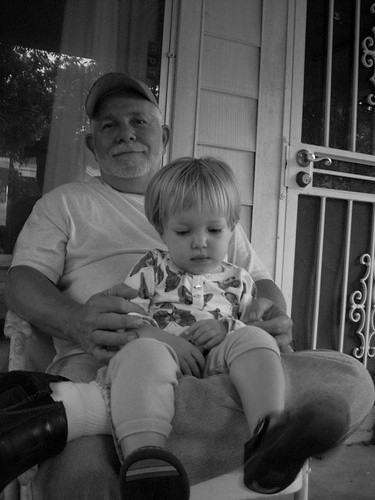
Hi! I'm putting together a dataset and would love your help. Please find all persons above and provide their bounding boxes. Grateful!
[0,71,375,500]
[99,151,353,500]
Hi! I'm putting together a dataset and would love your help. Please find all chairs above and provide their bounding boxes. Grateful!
[3,304,310,500]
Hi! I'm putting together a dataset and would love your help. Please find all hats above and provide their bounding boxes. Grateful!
[86,70,162,122]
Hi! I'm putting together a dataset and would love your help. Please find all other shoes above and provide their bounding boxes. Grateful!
[119,442,192,500]
[0,370,78,493]
[243,387,352,495]
